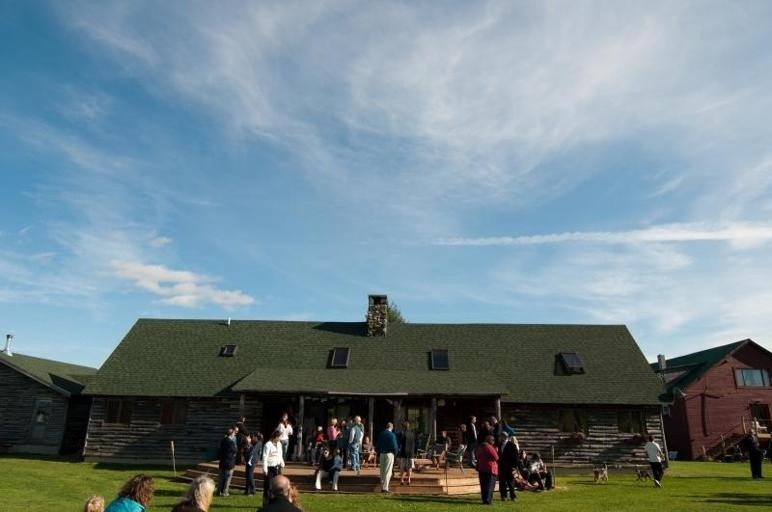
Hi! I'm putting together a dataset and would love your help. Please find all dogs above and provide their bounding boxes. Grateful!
[593,464,609,483]
[632,465,652,482]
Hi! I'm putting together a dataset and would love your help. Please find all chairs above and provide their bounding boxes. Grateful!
[445,443,467,474]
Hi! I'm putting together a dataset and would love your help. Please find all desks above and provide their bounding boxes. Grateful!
[360,451,377,470]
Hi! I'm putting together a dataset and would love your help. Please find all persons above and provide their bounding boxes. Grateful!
[255,476,303,511]
[643,434,666,487]
[748,427,765,478]
[170,472,216,512]
[104,472,155,511]
[84,494,106,511]
[220,412,554,504]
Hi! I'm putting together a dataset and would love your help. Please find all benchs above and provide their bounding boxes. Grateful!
[412,458,445,472]
